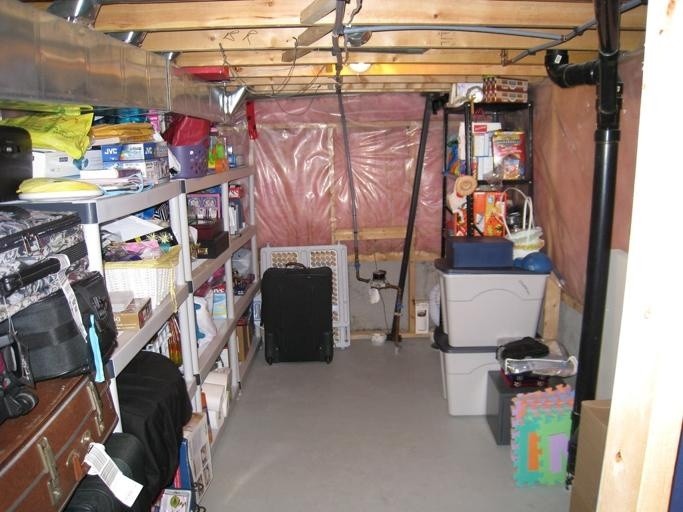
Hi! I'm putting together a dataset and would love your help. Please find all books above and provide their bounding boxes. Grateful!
[80,168,141,179]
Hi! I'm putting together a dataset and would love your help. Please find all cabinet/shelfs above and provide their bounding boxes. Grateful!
[0,178,204,434]
[179,127,262,449]
[431,99,534,349]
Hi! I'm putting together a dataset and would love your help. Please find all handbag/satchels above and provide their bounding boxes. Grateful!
[494,339,577,378]
[499,336,550,361]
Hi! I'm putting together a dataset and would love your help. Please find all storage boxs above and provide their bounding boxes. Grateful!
[486,370,566,446]
[196,231,229,260]
[454,190,508,238]
[458,122,502,161]
[569,399,612,512]
[188,219,224,240]
[483,77,529,93]
[434,263,552,349]
[446,236,515,268]
[477,156,494,181]
[438,349,501,416]
[236,313,253,362]
[483,90,529,103]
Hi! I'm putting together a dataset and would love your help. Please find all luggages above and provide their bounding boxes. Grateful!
[61,431,149,512]
[0,207,90,324]
[0,334,37,427]
[0,364,119,512]
[0,126,33,203]
[259,259,335,365]
[0,270,120,383]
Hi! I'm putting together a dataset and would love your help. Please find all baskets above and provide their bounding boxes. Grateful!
[166,136,210,180]
[504,225,545,261]
